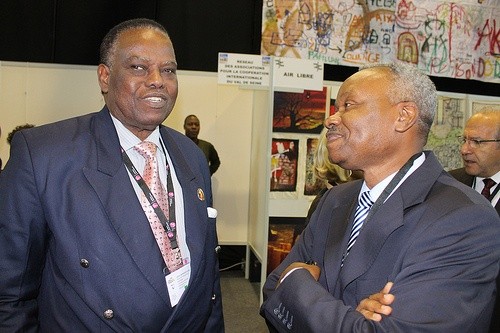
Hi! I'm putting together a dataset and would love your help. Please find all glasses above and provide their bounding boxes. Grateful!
[457,135,500,149]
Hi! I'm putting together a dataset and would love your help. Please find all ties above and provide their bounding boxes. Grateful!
[133,141,183,272]
[340,191,375,267]
[481,178,497,200]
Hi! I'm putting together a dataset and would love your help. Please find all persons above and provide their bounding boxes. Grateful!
[183,114,222,177]
[258,61,500,333]
[0,16,227,333]
[447,104,500,218]
